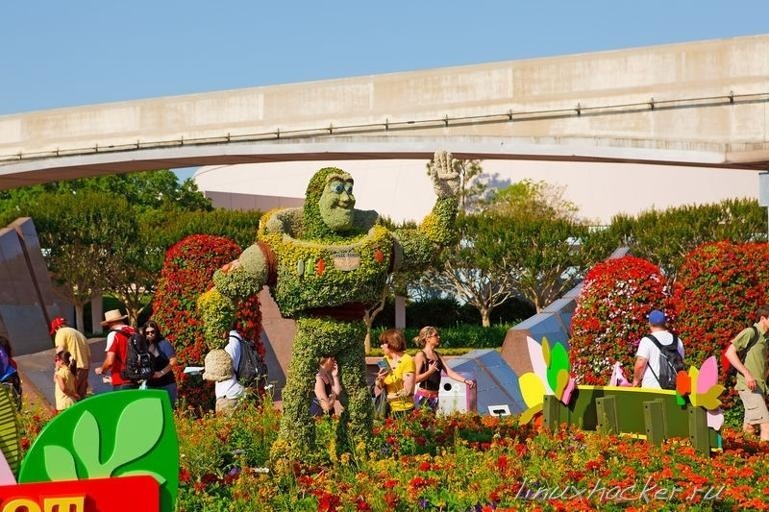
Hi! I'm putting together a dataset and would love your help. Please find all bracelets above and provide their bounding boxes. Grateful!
[463,378,470,383]
[158,368,167,377]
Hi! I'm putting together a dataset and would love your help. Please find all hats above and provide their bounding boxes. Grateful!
[99,309,127,326]
[49,317,64,336]
[646,310,665,325]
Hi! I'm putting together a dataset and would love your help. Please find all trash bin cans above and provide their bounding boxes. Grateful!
[438,372,477,412]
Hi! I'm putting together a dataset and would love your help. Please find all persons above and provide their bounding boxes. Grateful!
[724,304,769,442]
[0,335,24,412]
[54,350,81,414]
[198,148,463,472]
[48,315,91,401]
[632,309,684,390]
[93,307,139,389]
[213,319,246,417]
[310,352,343,417]
[374,327,416,419]
[409,323,478,413]
[142,319,179,410]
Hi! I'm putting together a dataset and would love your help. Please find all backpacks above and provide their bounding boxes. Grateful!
[643,332,683,389]
[106,329,155,380]
[227,335,267,388]
[728,326,757,375]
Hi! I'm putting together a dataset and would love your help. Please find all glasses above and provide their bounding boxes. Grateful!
[144,330,154,335]
[427,334,438,337]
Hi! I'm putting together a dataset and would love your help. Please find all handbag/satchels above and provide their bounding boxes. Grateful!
[309,399,323,417]
[418,396,430,406]
